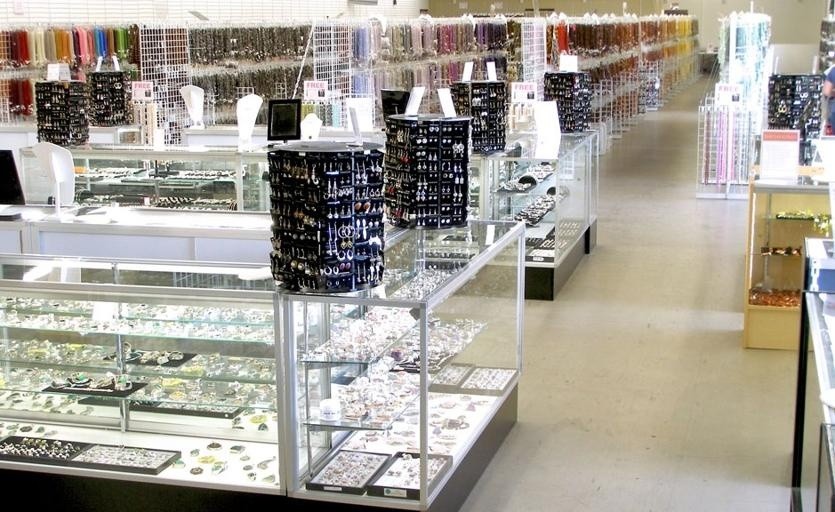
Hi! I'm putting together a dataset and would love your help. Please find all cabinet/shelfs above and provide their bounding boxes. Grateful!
[741,179,833,349]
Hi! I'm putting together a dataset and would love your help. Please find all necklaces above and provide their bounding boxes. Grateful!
[0,17,699,126]
